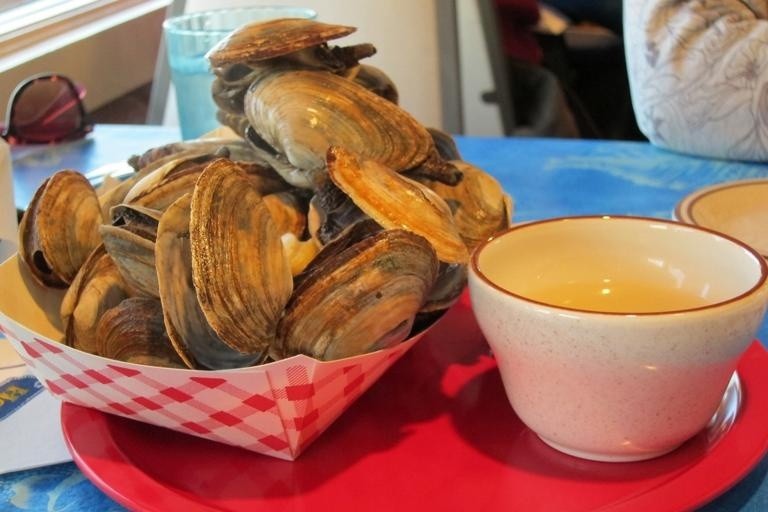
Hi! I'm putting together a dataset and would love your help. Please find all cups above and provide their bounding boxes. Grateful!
[463,215,768,463]
[161,6,319,139]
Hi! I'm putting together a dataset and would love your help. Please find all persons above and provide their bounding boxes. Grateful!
[623,0,766,163]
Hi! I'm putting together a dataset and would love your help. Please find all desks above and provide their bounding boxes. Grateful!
[0,125,768,510]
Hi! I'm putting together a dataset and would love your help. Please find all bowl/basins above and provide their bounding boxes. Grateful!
[671,178,768,263]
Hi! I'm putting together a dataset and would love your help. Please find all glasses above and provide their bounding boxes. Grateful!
[1,71,97,145]
